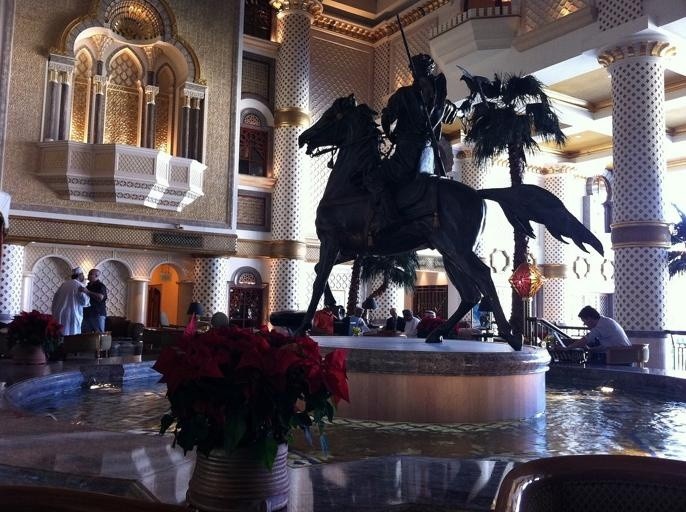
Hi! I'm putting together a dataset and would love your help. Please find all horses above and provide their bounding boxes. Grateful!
[291,93,604,351]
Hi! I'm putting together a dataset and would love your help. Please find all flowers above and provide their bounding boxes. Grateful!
[153,312,353,473]
[6,309,66,358]
[416,317,460,341]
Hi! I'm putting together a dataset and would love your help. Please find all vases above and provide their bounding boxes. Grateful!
[11,341,49,366]
[183,433,291,512]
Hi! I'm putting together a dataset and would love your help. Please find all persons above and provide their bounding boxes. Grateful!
[78,269,108,333]
[311,290,437,339]
[567,305,635,367]
[361,53,447,223]
[52,267,91,336]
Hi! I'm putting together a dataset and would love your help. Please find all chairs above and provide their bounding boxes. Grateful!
[494,455,685,512]
[549,342,651,370]
[63,330,113,359]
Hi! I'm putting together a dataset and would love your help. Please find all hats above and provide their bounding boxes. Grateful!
[73,267,82,275]
